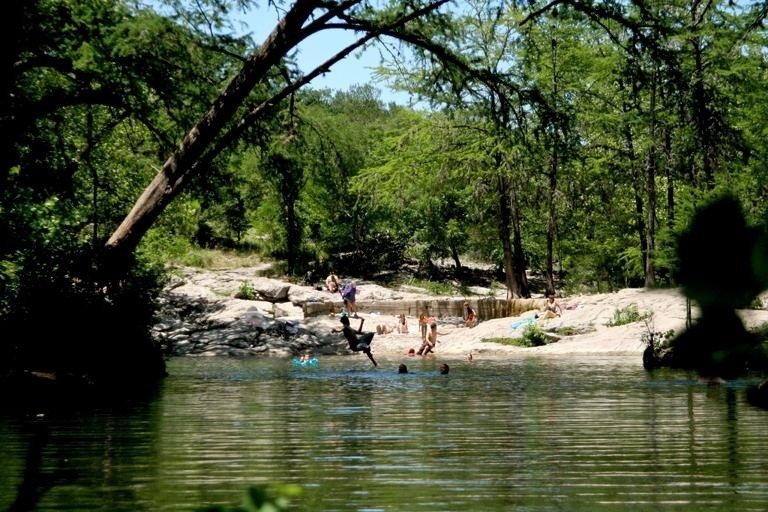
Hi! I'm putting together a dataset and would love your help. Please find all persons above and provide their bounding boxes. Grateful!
[440,364,450,374]
[537,296,563,321]
[377,311,454,357]
[325,269,360,318]
[330,314,379,366]
[463,352,474,363]
[464,302,477,327]
[398,364,408,374]
